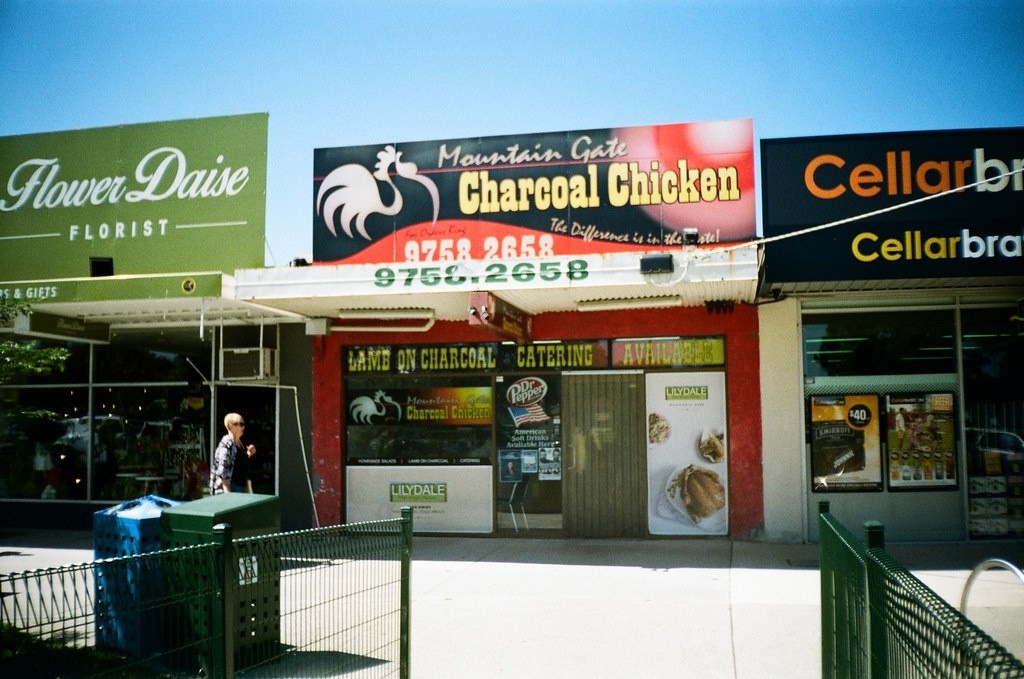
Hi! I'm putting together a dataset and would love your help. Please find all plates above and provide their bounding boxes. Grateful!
[665,464,726,533]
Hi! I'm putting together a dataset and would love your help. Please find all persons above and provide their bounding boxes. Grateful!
[369,417,401,461]
[208,413,257,496]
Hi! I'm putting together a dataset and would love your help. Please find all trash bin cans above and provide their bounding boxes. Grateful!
[160,492,282,676]
[93,493,191,661]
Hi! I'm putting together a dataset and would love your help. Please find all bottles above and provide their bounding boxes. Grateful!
[902,458,911,480]
[891,459,899,481]
[923,458,934,480]
[936,458,944,480]
[913,459,922,480]
[946,458,955,480]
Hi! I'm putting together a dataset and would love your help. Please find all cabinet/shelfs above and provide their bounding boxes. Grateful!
[965,428,1023,538]
[62,417,206,499]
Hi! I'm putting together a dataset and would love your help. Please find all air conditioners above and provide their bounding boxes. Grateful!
[219,348,271,380]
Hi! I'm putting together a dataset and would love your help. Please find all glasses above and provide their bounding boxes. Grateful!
[233,422,245,427]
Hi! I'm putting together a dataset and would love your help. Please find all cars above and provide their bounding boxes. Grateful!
[966,426,1024,468]
